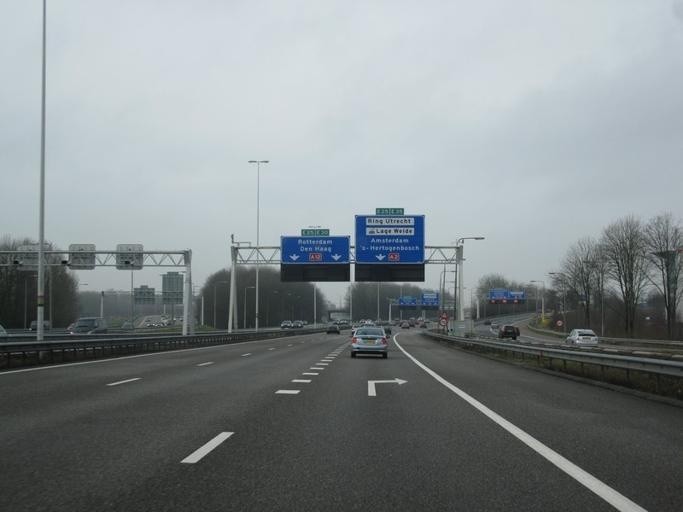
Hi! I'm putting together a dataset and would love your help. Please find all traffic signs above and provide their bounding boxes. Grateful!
[399,292,439,306]
[489,288,525,300]
[355,208,425,265]
[280,229,349,265]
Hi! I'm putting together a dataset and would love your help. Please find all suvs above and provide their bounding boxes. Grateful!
[29,321,49,330]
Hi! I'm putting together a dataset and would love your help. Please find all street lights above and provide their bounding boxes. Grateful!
[192,290,204,326]
[214,281,229,329]
[469,287,482,319]
[244,286,255,328]
[454,236,484,320]
[249,161,269,330]
[531,280,545,320]
[549,273,565,334]
[24,274,39,330]
[584,261,604,337]
[439,270,456,329]
[448,286,465,321]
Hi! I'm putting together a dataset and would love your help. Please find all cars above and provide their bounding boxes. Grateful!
[327,320,348,334]
[351,319,392,359]
[144,315,177,327]
[498,326,520,340]
[280,320,308,327]
[485,320,498,328]
[66,317,134,334]
[565,328,599,347]
[395,317,430,329]
